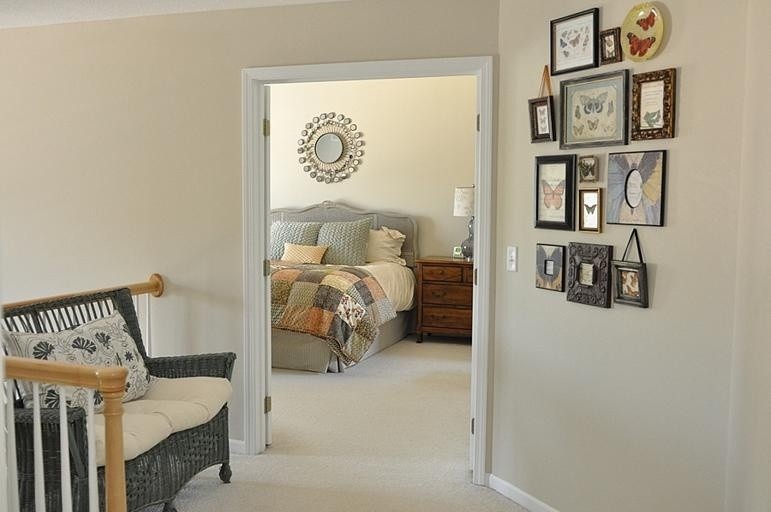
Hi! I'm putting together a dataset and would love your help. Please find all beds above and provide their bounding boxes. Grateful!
[270,200,418,373]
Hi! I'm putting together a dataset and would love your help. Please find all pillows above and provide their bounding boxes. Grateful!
[269,220,406,264]
[3,310,148,410]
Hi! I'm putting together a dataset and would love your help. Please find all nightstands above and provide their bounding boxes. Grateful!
[415,258,472,343]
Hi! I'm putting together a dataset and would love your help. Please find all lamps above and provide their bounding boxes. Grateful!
[454,187,474,260]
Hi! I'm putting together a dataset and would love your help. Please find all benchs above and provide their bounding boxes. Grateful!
[0,288,236,512]
